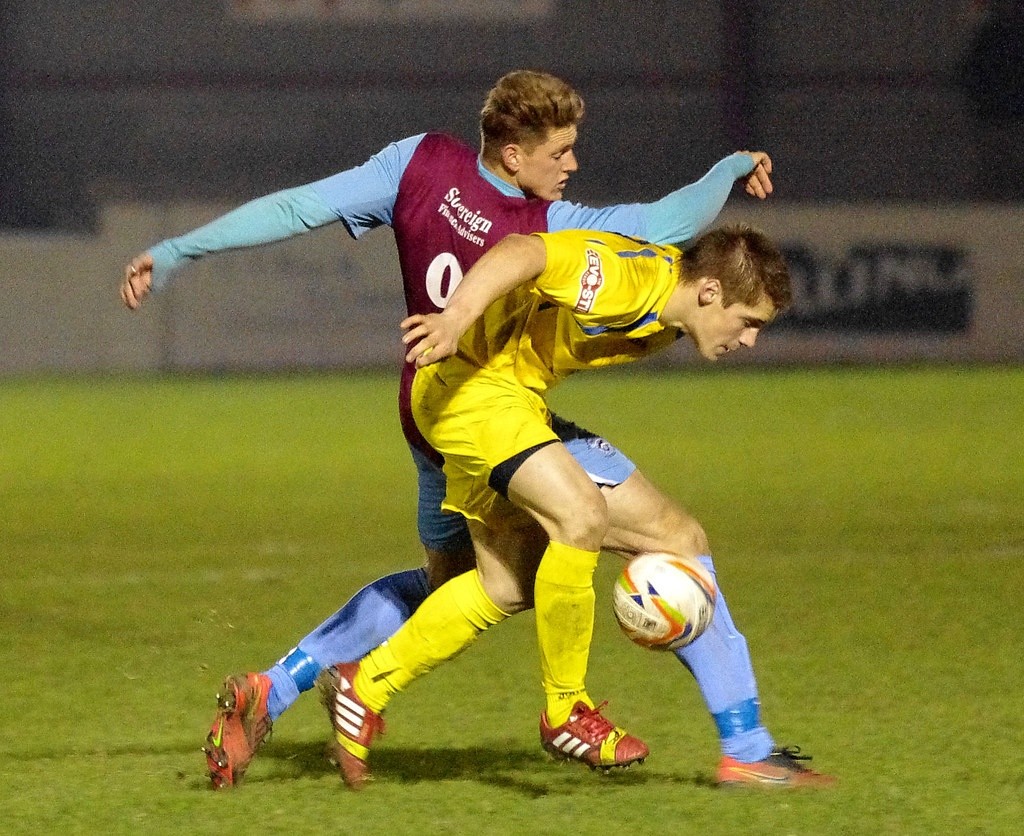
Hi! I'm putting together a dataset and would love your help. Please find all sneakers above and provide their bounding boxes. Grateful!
[202,673,272,794]
[320,661,386,792]
[715,747,837,794]
[539,701,650,775]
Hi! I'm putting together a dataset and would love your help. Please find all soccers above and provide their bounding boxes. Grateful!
[611,551,717,652]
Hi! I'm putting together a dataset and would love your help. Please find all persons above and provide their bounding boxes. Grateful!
[318,220,793,793]
[118,69,840,791]
